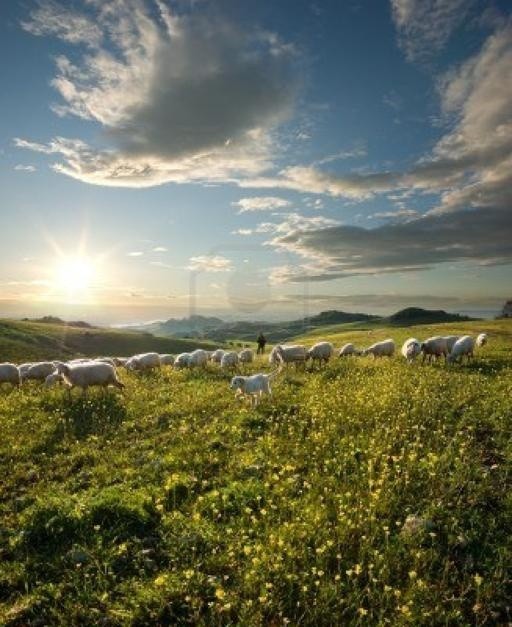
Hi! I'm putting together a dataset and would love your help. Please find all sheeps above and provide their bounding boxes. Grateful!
[340,339,395,361]
[0,351,175,398]
[177,348,255,372]
[402,336,474,366]
[477,333,488,349]
[272,341,332,374]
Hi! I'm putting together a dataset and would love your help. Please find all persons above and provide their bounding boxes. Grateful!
[256,329,266,354]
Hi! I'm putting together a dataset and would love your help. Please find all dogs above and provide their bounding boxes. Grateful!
[230,373,272,406]
